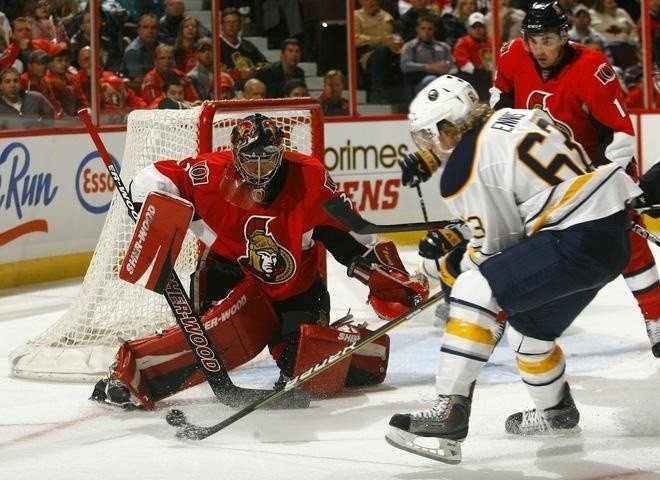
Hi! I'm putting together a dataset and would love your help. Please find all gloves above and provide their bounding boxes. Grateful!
[439,246,466,304]
[418,228,464,259]
[401,149,441,187]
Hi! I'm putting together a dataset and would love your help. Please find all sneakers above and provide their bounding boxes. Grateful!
[644,319,659,358]
[89,377,144,411]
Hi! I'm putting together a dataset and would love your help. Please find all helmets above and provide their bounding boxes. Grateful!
[518,2,569,71]
[230,114,286,188]
[407,74,480,161]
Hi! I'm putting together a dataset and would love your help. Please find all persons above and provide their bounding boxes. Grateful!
[486,3,660,358]
[397,69,505,354]
[1,1,660,132]
[387,69,644,443]
[92,110,431,410]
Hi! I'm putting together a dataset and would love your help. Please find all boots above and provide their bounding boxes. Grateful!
[506,381,580,434]
[388,379,476,442]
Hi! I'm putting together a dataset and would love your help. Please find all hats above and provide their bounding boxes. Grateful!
[467,12,486,26]
[193,36,212,51]
[27,49,53,63]
[572,4,589,16]
[47,41,68,57]
[211,72,234,90]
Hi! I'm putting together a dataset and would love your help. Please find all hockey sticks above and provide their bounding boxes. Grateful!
[165,291,449,440]
[78,108,310,409]
